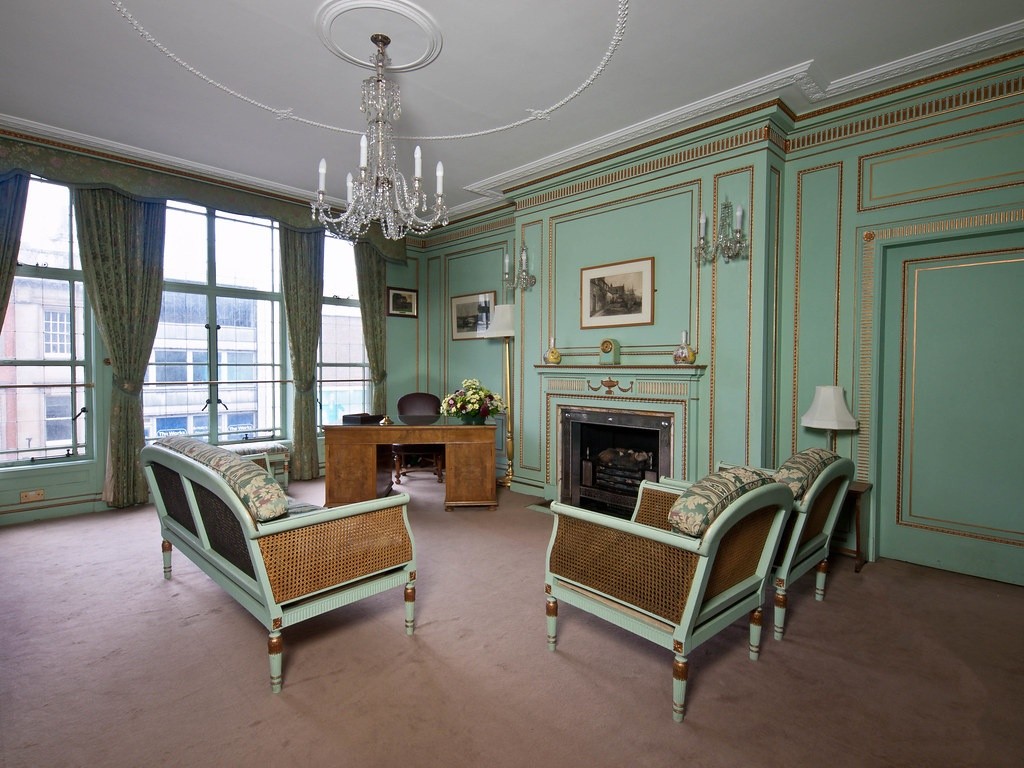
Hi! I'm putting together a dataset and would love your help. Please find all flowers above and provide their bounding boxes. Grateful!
[441,377,508,417]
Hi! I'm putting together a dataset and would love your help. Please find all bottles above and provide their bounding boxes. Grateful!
[543,337,561,365]
[674,330,696,364]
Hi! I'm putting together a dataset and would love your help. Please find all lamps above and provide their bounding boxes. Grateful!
[313,0,449,240]
[501,246,535,293]
[693,195,751,265]
[802,386,859,456]
[483,304,517,487]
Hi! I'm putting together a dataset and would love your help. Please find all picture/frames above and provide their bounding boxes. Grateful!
[580,258,654,331]
[388,286,419,317]
[451,291,497,340]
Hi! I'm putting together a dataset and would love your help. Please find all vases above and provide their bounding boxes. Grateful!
[672,329,695,363]
[544,337,561,364]
[460,408,488,426]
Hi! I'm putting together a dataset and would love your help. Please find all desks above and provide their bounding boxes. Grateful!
[838,482,873,574]
[322,425,498,512]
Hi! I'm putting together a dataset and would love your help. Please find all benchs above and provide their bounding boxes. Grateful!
[216,443,291,493]
[140,434,418,693]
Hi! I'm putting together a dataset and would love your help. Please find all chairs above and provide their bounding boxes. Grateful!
[660,449,859,641]
[395,392,443,485]
[545,464,796,723]
